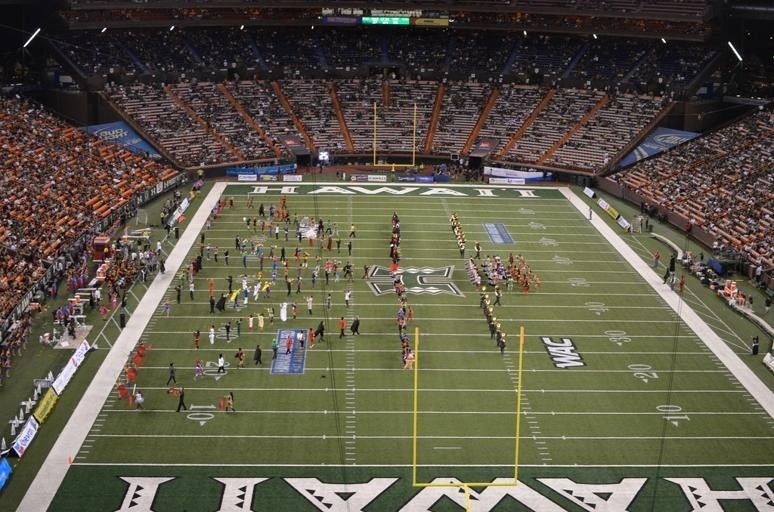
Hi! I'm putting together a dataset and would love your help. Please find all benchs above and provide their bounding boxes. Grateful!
[610,111,774,346]
[0,89,177,343]
[51,29,669,168]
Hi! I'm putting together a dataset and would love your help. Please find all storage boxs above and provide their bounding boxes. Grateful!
[610,111,774,346]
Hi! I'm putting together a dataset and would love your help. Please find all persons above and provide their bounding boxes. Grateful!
[0,0,774,412]
